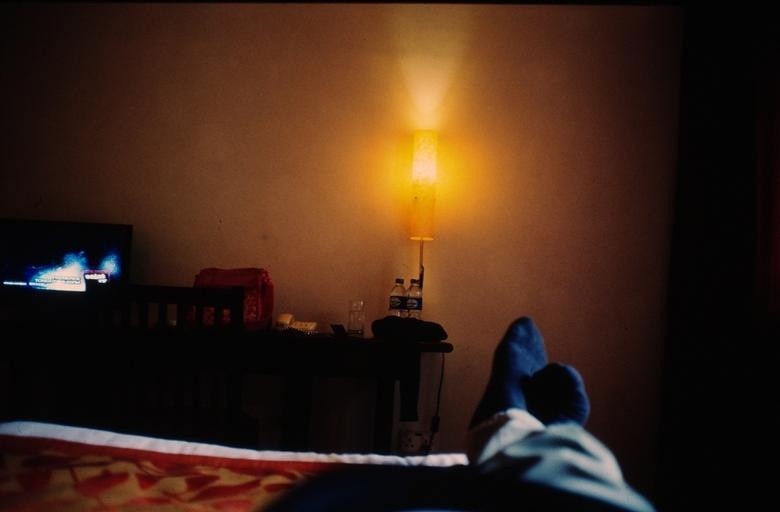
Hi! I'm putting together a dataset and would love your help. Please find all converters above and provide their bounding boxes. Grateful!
[431,416,440,433]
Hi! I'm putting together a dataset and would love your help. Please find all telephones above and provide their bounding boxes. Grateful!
[276,313,317,333]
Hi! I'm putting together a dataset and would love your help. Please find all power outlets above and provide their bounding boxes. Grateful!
[403,433,441,456]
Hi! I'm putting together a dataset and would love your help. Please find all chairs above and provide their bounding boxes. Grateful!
[85,280,265,448]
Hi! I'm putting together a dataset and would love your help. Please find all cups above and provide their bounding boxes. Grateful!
[346,298,364,335]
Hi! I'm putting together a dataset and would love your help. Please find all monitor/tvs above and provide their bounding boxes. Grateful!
[0,216,133,303]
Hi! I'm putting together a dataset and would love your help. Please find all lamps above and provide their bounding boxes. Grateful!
[407,128,443,319]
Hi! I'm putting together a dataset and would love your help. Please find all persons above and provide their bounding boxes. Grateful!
[466,315,656,512]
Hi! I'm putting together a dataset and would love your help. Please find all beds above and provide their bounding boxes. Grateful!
[1,421,470,512]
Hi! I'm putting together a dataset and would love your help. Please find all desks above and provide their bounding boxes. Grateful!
[0,325,453,454]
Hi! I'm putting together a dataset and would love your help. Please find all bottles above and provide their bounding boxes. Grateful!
[388,278,423,319]
